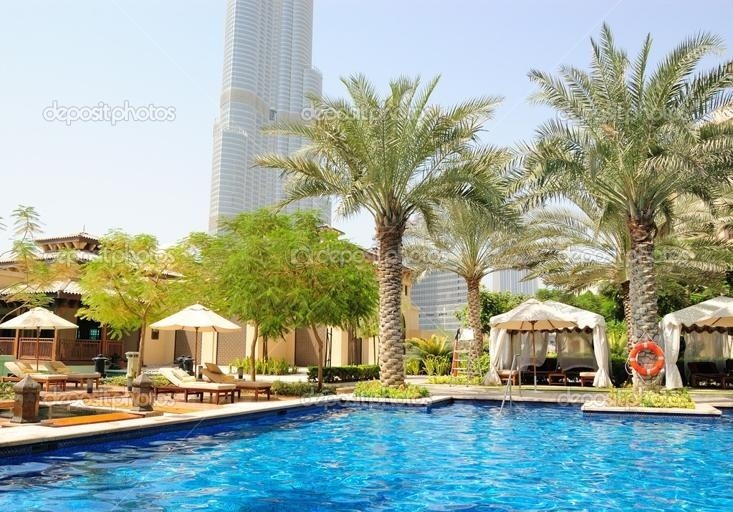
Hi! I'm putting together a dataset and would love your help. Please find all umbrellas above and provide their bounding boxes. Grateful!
[485,297,579,389]
[147,301,243,379]
[695,299,733,329]
[0,305,80,372]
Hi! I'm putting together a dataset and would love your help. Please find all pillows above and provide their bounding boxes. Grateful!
[205,362,221,373]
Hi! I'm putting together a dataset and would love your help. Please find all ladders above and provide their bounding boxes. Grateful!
[451,329,483,386]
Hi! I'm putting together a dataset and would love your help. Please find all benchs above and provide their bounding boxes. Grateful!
[518,357,593,385]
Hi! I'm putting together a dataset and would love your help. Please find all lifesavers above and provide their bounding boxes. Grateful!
[629,342,665,376]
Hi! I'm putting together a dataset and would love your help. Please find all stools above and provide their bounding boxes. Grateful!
[579,370,597,388]
[495,369,518,385]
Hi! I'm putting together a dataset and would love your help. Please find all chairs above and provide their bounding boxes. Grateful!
[154,368,272,405]
[3,360,101,392]
[687,361,731,389]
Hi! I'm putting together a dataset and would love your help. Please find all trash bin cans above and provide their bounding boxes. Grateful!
[125,351,140,377]
